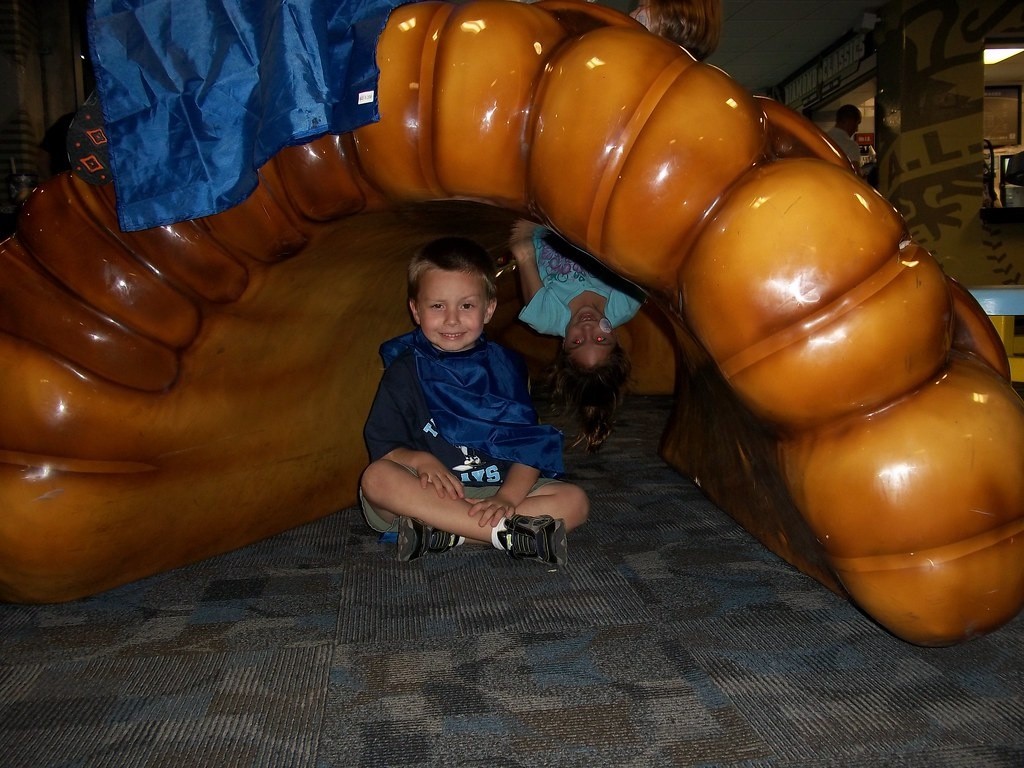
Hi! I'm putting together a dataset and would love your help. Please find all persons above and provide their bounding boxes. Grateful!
[509,218,643,449]
[826,104,862,175]
[359,236,588,568]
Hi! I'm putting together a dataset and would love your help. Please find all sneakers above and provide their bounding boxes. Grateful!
[496,513,570,566]
[396,516,460,563]
